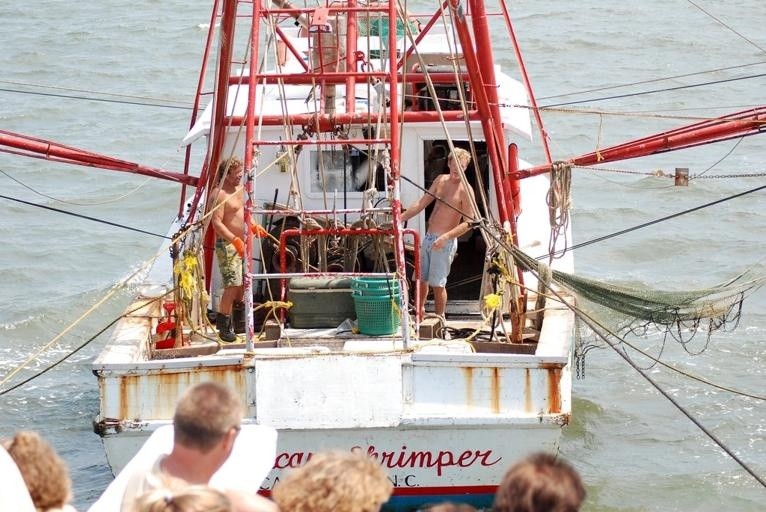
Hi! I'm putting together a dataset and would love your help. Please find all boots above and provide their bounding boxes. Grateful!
[233,307,261,333]
[217,312,236,342]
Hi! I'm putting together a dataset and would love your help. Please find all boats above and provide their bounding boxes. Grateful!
[1,2,765,511]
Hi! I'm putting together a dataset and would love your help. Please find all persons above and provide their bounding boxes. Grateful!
[2,429,81,512]
[120,382,265,511]
[271,450,394,511]
[208,159,269,344]
[149,482,233,512]
[380,145,477,325]
[493,452,588,512]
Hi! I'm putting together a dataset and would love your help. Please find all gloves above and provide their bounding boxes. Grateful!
[249,220,268,239]
[233,236,245,260]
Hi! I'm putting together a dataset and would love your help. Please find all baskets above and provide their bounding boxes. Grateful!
[349,274,403,336]
